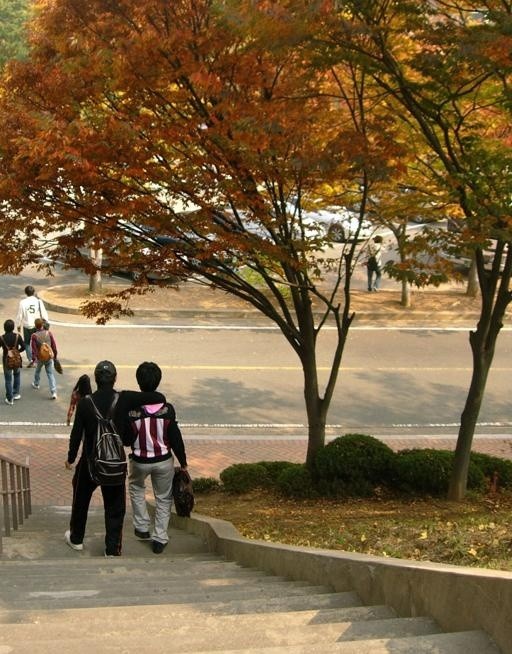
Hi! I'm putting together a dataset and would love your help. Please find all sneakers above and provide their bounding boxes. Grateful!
[5,398,13,405]
[13,394,21,400]
[63,529,83,551]
[152,539,168,554]
[103,545,122,558]
[51,392,57,399]
[31,382,39,389]
[134,529,151,539]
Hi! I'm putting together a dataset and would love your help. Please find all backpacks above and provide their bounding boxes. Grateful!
[367,244,381,273]
[84,393,128,487]
[32,330,54,362]
[0,333,22,370]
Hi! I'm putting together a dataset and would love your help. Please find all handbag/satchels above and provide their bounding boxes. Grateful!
[54,358,62,374]
[42,319,49,330]
[172,467,194,518]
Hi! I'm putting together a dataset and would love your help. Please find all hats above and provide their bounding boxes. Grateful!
[94,358,118,383]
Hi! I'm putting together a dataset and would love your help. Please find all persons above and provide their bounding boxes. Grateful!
[0,318,25,406]
[16,286,49,368]
[31,318,58,399]
[366,235,385,293]
[127,362,189,553]
[66,374,92,442]
[64,360,166,557]
